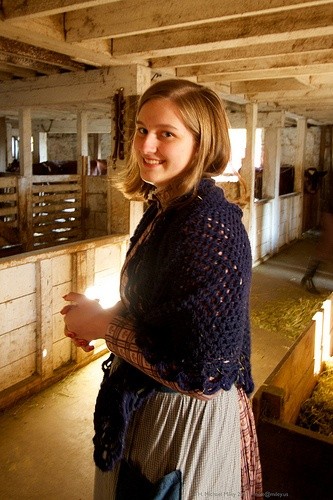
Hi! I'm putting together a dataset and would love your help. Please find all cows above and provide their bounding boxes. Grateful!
[235,164,328,200]
[5,159,107,196]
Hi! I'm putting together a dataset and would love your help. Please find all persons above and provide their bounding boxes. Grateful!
[61,78,264,499]
